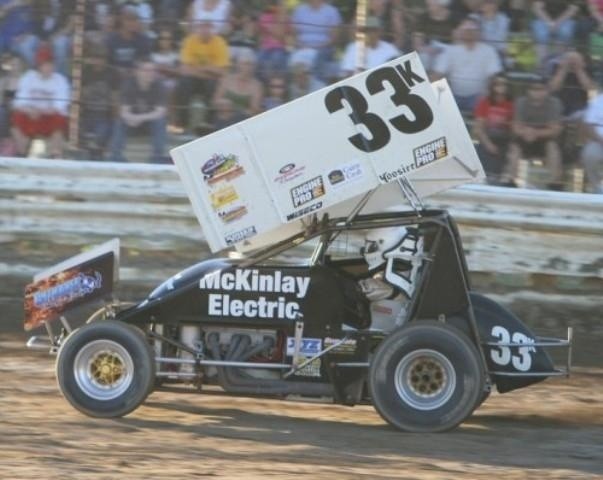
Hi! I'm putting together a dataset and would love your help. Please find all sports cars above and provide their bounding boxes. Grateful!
[21,205,576,434]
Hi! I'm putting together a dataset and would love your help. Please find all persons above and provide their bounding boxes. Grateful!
[1,1,603,195]
[328,225,411,332]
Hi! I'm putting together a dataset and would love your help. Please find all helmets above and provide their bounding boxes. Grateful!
[363,226,410,276]
